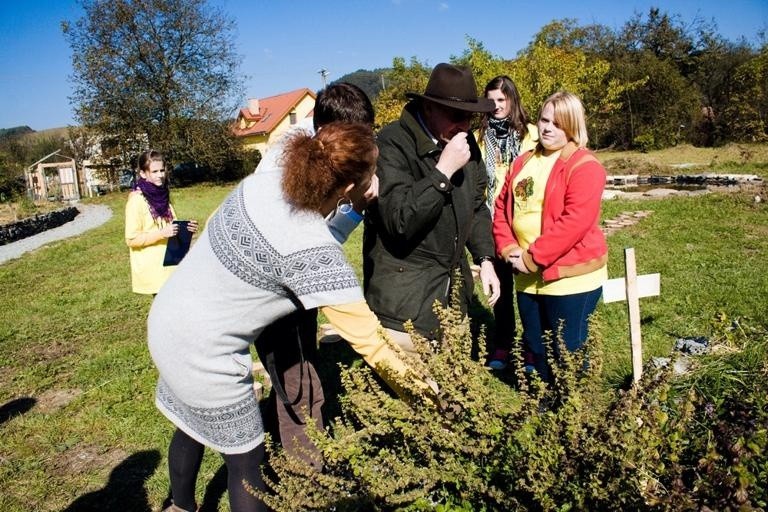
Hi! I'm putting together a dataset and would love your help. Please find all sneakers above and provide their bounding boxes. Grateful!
[162,499,200,512]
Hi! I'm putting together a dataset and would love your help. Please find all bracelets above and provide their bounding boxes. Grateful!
[479,257,495,266]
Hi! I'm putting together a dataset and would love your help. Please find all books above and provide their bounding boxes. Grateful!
[163,221,194,267]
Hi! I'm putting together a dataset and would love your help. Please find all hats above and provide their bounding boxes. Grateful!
[405,62,496,112]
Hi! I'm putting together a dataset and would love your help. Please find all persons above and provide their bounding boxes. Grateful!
[250,83,381,511]
[492,90,610,410]
[473,76,541,386]
[122,152,199,299]
[144,118,444,510]
[363,62,501,425]
